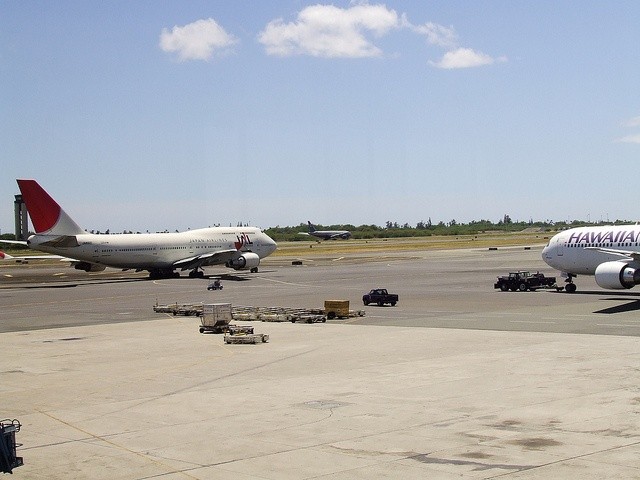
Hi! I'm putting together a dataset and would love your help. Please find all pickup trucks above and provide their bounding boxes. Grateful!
[363,289,398,306]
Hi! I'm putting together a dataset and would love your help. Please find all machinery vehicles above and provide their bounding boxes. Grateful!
[494,270,545,292]
[531,271,556,287]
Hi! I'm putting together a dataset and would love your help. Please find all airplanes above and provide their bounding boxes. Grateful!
[298,221,351,240]
[541,225,640,293]
[0,179,277,279]
